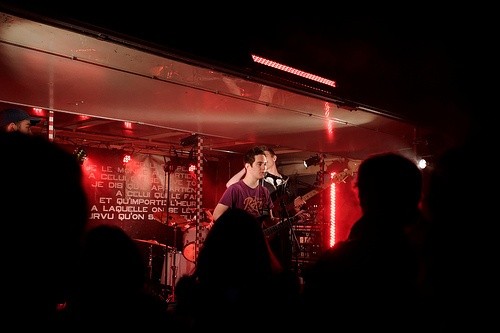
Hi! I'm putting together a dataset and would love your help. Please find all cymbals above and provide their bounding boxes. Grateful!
[131,238,175,250]
[152,213,191,229]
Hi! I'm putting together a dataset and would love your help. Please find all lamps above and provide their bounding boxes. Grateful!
[163,135,197,172]
[303,155,326,167]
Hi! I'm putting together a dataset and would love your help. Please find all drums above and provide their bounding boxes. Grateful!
[181,220,210,263]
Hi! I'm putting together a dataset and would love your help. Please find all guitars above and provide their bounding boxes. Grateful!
[259,203,327,238]
[262,167,354,230]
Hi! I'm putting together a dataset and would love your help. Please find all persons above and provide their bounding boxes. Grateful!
[0,108,32,135]
[0,130,500,333]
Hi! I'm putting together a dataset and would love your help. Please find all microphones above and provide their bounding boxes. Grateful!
[264,172,281,179]
